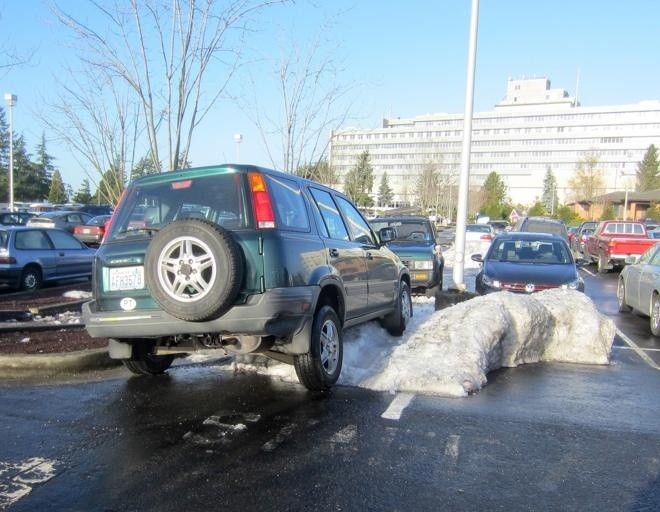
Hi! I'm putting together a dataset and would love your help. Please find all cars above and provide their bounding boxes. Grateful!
[466,215,660,336]
[0,201,151,292]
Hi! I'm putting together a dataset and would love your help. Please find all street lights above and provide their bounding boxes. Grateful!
[5,94,17,213]
[233,133,243,165]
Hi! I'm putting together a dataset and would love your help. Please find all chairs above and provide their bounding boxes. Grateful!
[501,242,553,261]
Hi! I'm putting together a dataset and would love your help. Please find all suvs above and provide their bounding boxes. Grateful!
[81,168,413,390]
[368,218,445,298]
